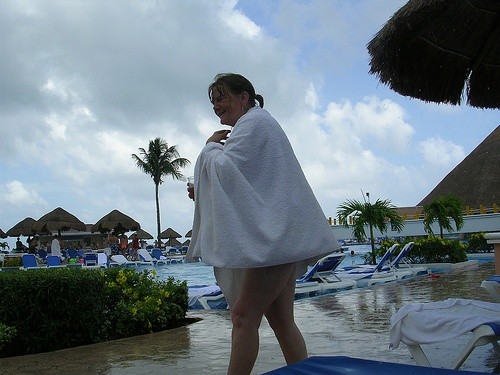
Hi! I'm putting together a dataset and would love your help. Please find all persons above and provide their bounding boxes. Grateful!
[187,72,341,375]
[153,239,158,247]
[15,237,24,250]
[27,236,99,253]
[130,234,140,255]
[350,251,357,256]
[120,234,128,256]
[106,235,119,254]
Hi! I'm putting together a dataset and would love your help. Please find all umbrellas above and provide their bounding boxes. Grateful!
[4,218,52,248]
[92,210,140,237]
[0,229,7,238]
[166,239,182,247]
[185,229,193,237]
[364,0,500,111]
[128,229,153,241]
[182,239,191,247]
[32,207,87,239]
[157,228,182,246]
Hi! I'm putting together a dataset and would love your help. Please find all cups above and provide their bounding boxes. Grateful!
[187,176,194,188]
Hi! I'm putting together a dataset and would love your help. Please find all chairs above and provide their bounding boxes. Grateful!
[151,246,168,259]
[166,247,176,255]
[181,246,189,254]
[295,253,357,298]
[258,353,493,375]
[349,240,427,279]
[478,274,500,302]
[340,243,399,286]
[388,298,500,369]
[137,248,157,262]
[67,244,83,258]
[85,254,97,265]
[46,255,60,267]
[187,283,229,310]
[21,253,38,269]
[110,254,128,263]
